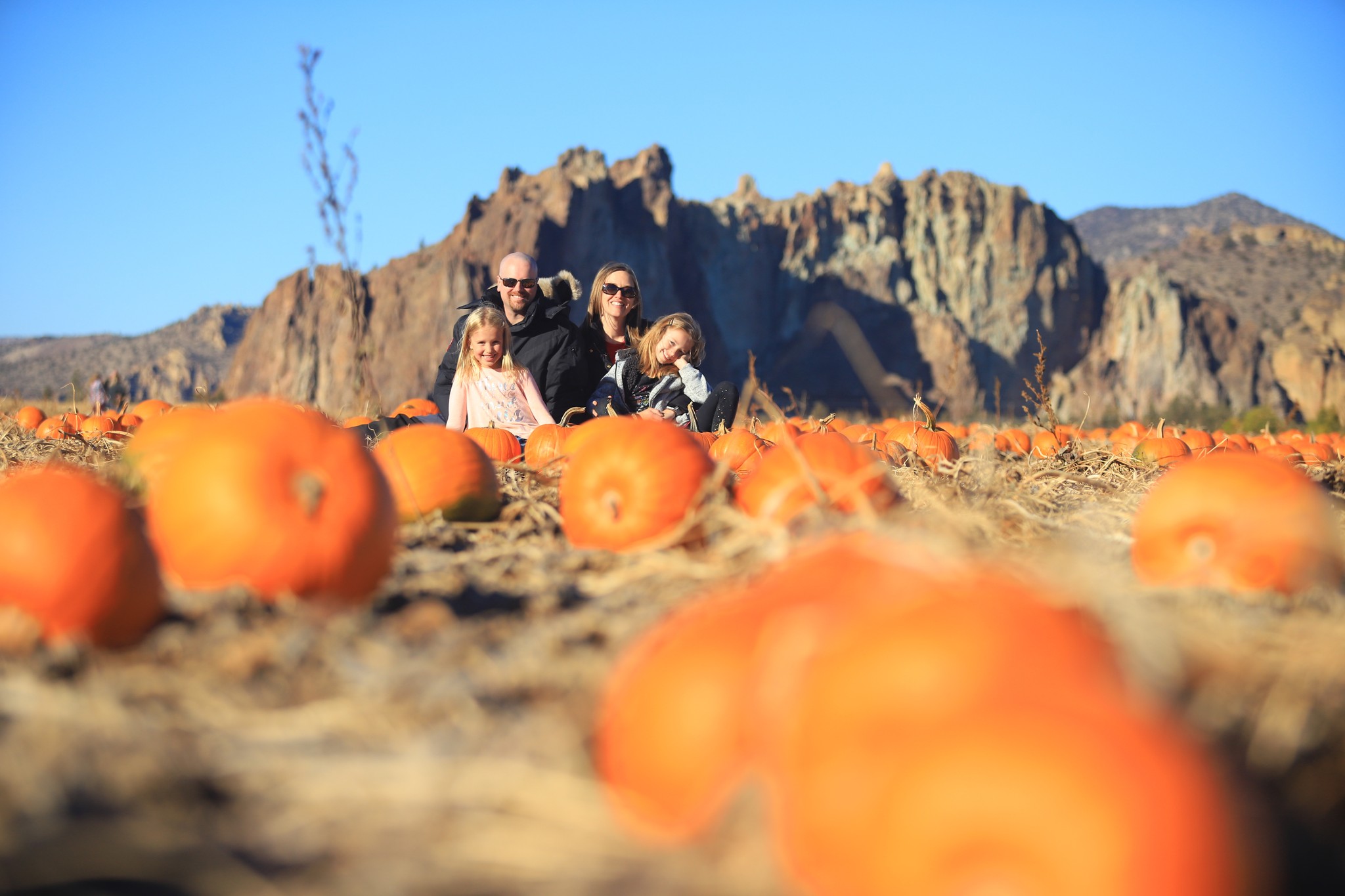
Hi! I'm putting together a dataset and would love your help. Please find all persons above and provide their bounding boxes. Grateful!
[344,251,738,463]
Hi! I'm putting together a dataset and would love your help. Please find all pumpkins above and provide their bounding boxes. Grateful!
[0,392,523,669]
[583,534,1282,896]
[523,401,1344,602]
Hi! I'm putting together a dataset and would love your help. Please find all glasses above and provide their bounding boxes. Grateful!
[500,277,538,288]
[603,283,637,298]
